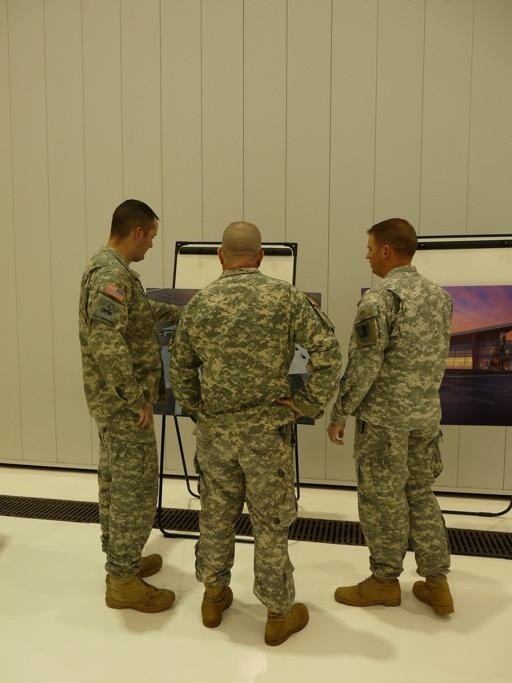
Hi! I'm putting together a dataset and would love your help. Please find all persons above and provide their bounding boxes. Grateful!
[78,198,184,613]
[168,220,344,647]
[326,216,458,618]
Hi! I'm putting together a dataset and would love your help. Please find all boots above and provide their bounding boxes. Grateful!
[104,553,163,579]
[201,586,234,629]
[264,603,309,646]
[334,574,401,607]
[105,572,175,612]
[412,576,454,615]
